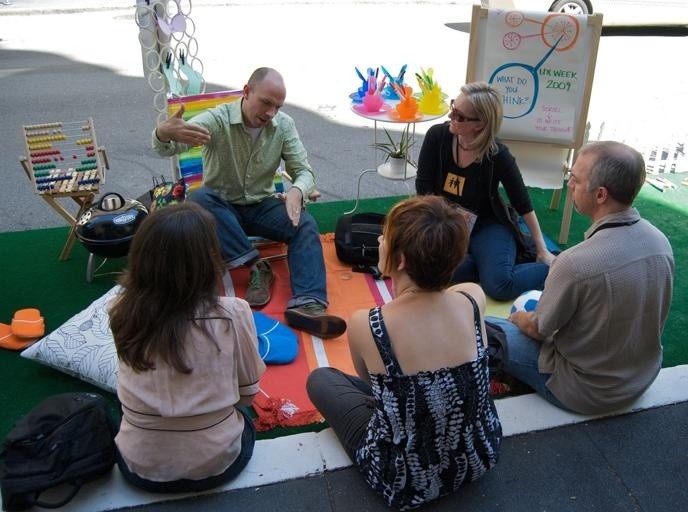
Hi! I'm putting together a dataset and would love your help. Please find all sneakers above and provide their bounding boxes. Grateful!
[285,303,347,339]
[245,261,275,305]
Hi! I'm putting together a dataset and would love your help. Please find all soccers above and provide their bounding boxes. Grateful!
[511,289,543,312]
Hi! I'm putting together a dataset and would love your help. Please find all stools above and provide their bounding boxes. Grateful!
[40,170,99,265]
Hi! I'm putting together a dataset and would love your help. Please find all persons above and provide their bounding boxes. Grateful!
[415,80,566,301]
[484,142,676,416]
[306,193,503,511]
[151,68,346,340]
[110,200,266,493]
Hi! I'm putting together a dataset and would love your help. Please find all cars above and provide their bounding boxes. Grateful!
[479,0,687,37]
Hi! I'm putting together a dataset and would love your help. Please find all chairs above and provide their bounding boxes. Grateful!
[164,89,289,267]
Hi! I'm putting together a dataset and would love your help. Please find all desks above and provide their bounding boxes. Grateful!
[344,93,449,217]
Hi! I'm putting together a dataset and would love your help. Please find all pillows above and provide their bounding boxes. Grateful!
[20,283,127,394]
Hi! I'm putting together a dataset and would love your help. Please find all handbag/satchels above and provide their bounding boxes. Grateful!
[513,215,562,259]
[0,392,116,511]
[335,212,385,266]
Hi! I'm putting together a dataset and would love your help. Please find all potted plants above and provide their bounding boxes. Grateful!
[368,127,420,174]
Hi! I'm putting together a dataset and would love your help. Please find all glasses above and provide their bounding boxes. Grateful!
[450,99,481,122]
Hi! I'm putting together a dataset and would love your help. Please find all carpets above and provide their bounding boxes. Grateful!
[0,167,688,443]
